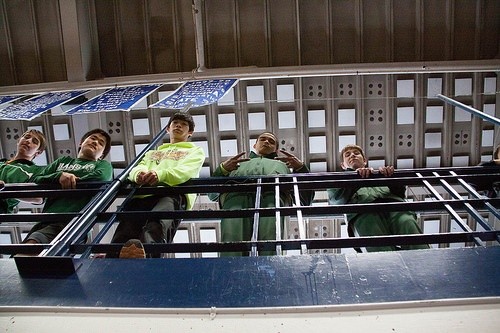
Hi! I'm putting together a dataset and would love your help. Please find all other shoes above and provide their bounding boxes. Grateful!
[120,239,146,259]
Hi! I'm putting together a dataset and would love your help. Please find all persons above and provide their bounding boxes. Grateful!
[462,142,500,209]
[327,144,434,252]
[208,132,315,258]
[14,129,114,257]
[0,129,46,226]
[105,111,206,258]
